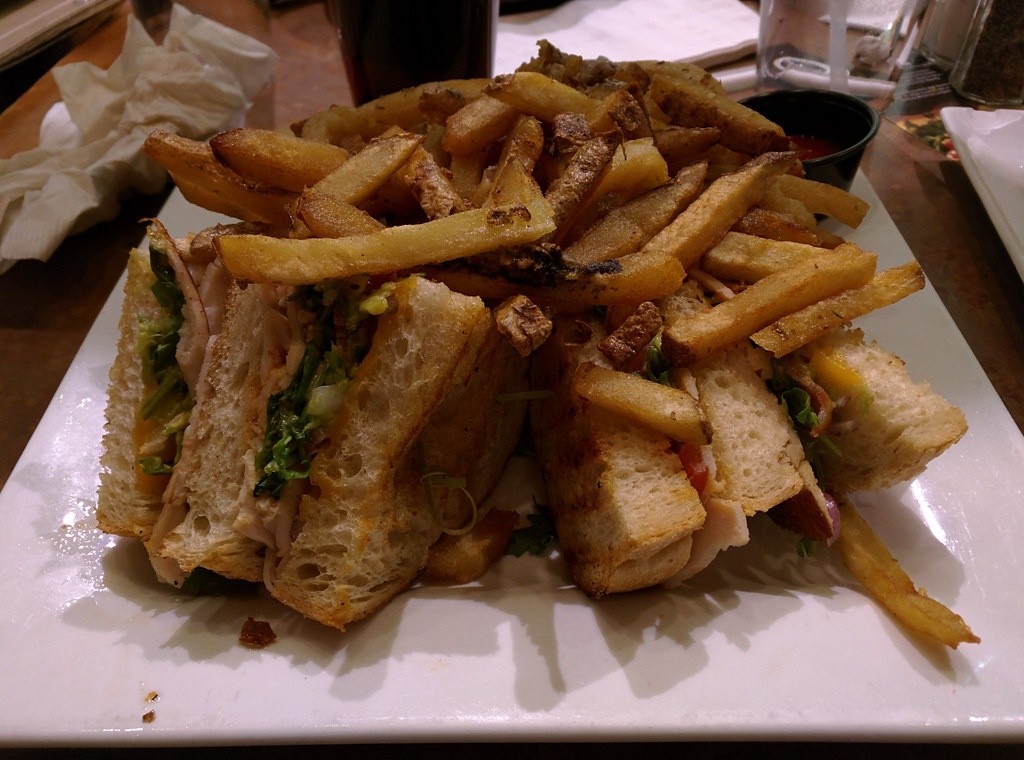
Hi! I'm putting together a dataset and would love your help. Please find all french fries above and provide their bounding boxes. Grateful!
[132,39,928,439]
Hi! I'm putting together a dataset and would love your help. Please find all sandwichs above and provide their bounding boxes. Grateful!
[103,225,966,636]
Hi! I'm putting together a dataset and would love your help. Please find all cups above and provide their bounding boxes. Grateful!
[324,0,500,124]
[756,0,931,115]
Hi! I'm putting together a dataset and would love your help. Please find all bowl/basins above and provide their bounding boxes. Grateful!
[735,88,880,222]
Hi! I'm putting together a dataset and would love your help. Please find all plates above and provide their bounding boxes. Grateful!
[0,107,1024,748]
